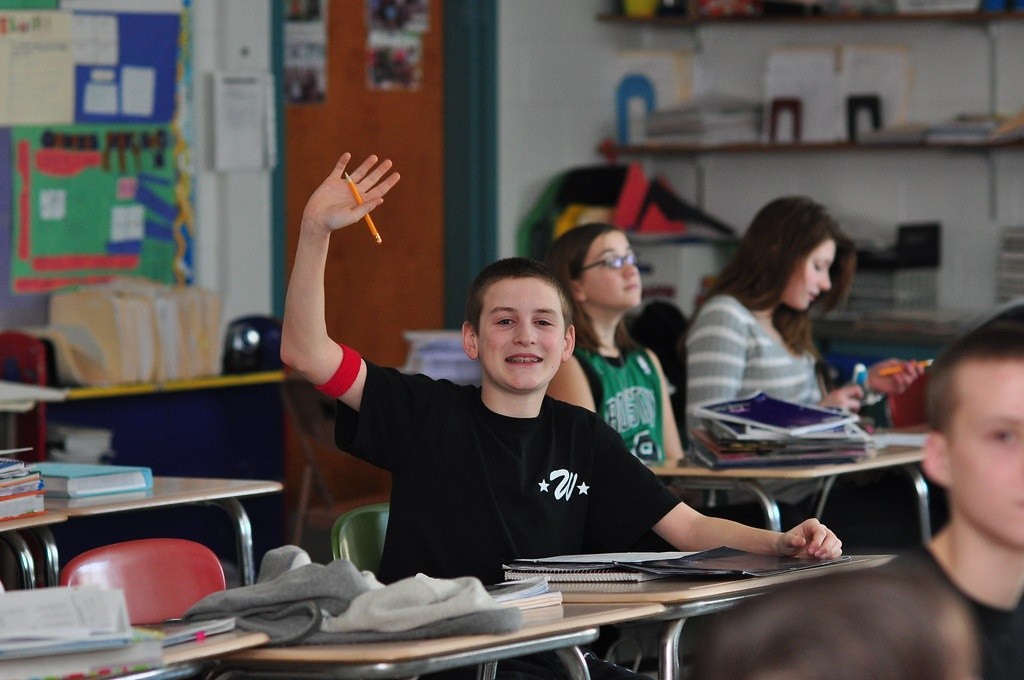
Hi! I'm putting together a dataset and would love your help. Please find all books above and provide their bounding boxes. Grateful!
[487,576,563,610]
[648,101,764,148]
[927,112,1016,145]
[501,546,852,583]
[683,391,875,470]
[0,458,236,680]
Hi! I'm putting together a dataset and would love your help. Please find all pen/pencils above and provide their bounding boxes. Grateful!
[341,171,384,246]
[875,357,934,376]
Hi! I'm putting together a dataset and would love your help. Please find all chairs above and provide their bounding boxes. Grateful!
[58,538,226,624]
[330,502,392,575]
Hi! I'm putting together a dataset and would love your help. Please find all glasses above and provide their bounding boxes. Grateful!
[580,253,637,269]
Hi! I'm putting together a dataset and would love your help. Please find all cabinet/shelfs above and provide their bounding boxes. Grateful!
[592,8,1024,344]
[0,324,287,584]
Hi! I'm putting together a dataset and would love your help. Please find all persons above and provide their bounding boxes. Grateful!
[280,153,842,680]
[685,193,925,552]
[695,566,980,680]
[883,316,1024,680]
[547,222,796,534]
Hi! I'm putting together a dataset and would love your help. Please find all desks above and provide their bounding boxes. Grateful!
[0,424,931,680]
